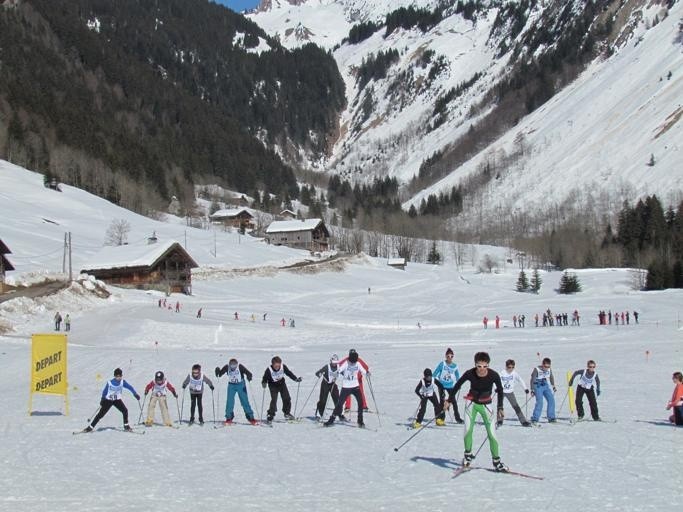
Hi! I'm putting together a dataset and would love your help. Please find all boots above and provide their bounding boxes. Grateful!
[462,452,475,467]
[493,457,509,472]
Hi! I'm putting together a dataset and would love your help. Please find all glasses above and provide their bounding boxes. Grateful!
[475,364,488,369]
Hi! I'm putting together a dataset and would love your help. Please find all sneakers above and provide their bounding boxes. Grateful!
[314,407,373,428]
[224,418,232,425]
[123,424,131,432]
[411,413,602,429]
[83,425,92,432]
[283,412,295,420]
[249,419,258,425]
[145,417,204,427]
[266,412,273,421]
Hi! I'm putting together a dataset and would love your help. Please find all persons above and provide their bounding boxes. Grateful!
[64,315,71,331]
[54,312,62,331]
[368,288,371,295]
[85,368,140,433]
[159,298,295,327]
[144,371,178,427]
[442,352,506,472]
[483,309,640,329]
[666,372,683,426]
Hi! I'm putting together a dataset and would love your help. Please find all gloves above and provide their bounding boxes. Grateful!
[496,407,504,426]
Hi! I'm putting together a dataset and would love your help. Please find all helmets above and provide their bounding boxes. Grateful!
[155,371,163,382]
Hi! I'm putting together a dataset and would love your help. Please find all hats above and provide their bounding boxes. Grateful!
[329,349,358,363]
[113,368,122,377]
